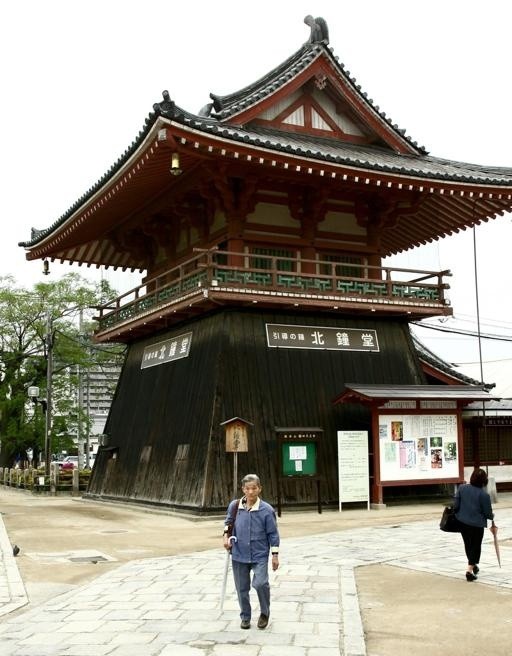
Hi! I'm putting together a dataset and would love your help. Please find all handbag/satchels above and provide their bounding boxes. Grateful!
[440,508,461,533]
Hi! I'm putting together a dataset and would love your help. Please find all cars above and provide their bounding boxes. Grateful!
[50,452,97,471]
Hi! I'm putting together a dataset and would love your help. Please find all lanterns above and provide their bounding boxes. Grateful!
[165,150,184,176]
[40,260,52,276]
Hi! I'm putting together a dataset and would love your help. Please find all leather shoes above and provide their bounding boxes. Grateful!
[258,613,268,628]
[241,622,250,629]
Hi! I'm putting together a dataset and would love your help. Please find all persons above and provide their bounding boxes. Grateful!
[450,469,494,581]
[223,474,280,628]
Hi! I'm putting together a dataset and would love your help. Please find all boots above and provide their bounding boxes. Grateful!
[466,566,479,581]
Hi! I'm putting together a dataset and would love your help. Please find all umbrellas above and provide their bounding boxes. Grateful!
[218,536,237,620]
[489,519,503,569]
